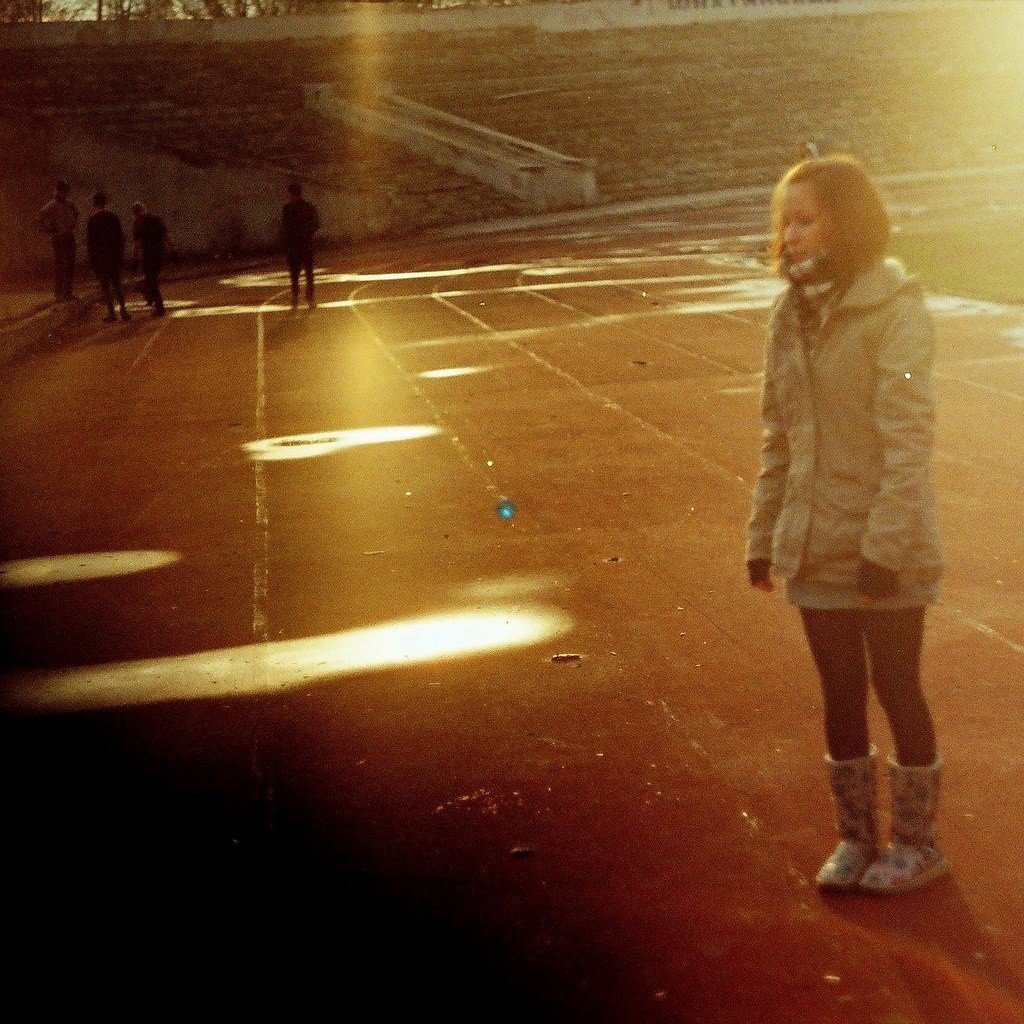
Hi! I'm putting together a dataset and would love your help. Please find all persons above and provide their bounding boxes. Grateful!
[744,156,947,893]
[131,203,168,315]
[86,193,131,321]
[279,184,320,307]
[37,181,83,299]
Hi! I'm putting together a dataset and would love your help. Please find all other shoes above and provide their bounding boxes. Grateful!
[121,312,133,321]
[102,312,117,323]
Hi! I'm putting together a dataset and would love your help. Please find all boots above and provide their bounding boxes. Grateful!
[856,748,950,895]
[815,742,880,889]
